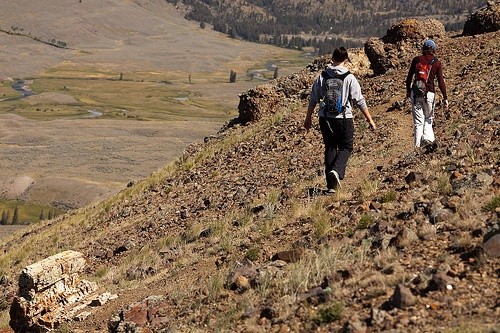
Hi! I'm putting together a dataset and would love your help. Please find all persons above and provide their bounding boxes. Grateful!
[302,46,377,193]
[403,39,448,153]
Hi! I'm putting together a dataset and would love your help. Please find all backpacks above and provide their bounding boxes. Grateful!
[412,56,438,97]
[321,70,352,115]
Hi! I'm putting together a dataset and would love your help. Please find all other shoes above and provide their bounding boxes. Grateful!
[326,188,336,195]
[329,169,342,187]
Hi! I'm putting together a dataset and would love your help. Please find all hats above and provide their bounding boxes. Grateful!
[424,39,435,49]
